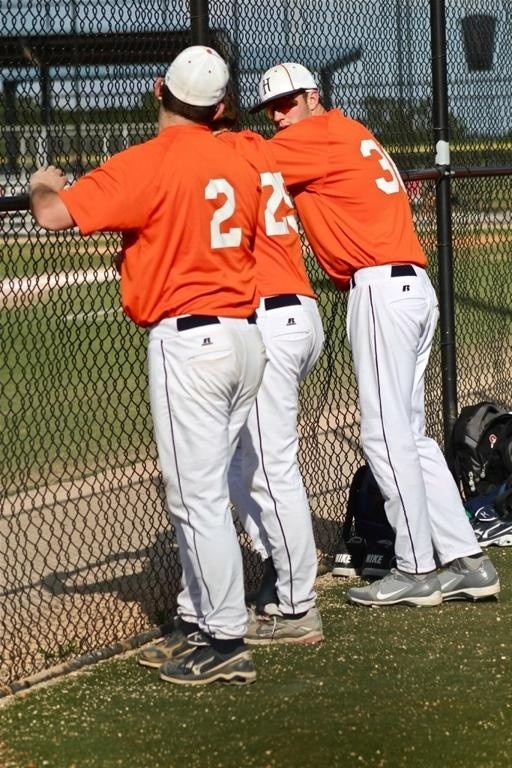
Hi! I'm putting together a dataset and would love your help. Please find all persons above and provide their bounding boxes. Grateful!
[249,60,502,608]
[29,44,262,686]
[214,73,328,649]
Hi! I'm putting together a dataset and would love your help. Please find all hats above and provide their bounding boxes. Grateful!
[165,45,229,107]
[249,63,318,113]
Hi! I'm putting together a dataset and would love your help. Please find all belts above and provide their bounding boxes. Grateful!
[352,265,416,287]
[265,295,300,310]
[177,315,255,331]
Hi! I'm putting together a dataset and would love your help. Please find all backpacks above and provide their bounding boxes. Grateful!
[342,461,395,539]
[448,401,512,511]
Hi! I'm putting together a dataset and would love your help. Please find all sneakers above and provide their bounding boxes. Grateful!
[474,506,512,547]
[139,631,257,686]
[243,597,325,645]
[332,544,500,607]
